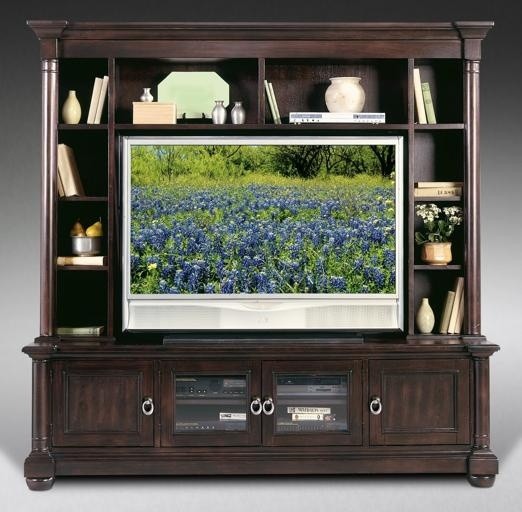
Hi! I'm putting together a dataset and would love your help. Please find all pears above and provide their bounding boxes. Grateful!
[86,217,104,236]
[70,218,85,237]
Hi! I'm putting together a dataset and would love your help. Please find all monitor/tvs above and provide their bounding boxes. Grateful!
[117,133,405,344]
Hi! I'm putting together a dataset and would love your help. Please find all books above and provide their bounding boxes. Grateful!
[86,75,110,125]
[56,144,85,197]
[54,325,104,337]
[57,257,106,267]
[413,182,462,198]
[440,277,467,335]
[413,67,437,125]
[265,79,282,125]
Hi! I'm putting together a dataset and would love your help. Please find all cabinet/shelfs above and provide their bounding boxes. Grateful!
[22,27,502,493]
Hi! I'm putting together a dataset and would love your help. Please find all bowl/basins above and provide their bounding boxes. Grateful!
[70,236,103,256]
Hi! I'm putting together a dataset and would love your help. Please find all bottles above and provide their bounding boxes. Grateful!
[325,77,366,112]
[140,87,154,102]
[212,100,246,124]
[416,298,436,333]
[62,90,81,125]
